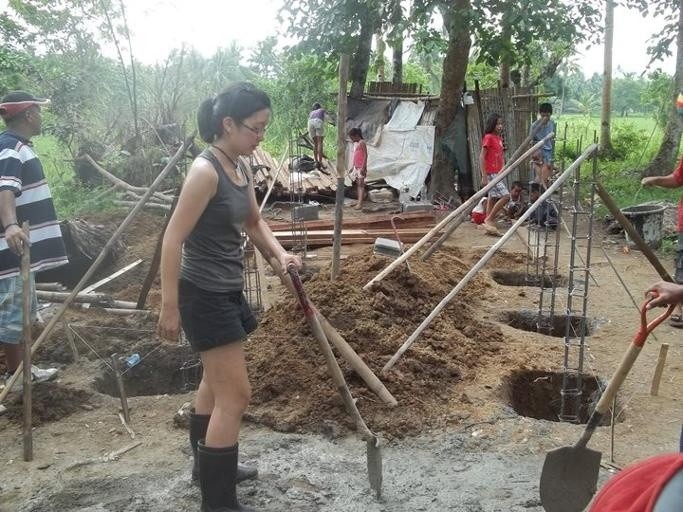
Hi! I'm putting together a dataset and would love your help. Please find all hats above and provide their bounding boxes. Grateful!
[0,90,51,121]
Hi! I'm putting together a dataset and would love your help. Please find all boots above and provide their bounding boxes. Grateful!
[190,414,258,484]
[196,437,256,512]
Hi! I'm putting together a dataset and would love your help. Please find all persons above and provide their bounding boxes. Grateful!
[0,94,69,390]
[530,103,558,187]
[347,126,367,211]
[152,80,303,511]
[639,90,682,330]
[478,114,512,236]
[471,188,487,225]
[526,181,560,229]
[584,452,682,512]
[505,181,525,219]
[643,281,683,310]
[306,101,328,170]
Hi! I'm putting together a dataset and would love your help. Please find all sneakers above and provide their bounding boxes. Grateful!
[669,314,683,327]
[3,364,59,393]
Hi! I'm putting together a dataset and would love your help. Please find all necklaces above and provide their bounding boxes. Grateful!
[211,144,239,169]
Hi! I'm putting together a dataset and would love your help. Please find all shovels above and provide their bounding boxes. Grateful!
[539,291,677,512]
[287,262,382,501]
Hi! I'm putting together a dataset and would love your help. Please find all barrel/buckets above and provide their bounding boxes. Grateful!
[620,203,668,251]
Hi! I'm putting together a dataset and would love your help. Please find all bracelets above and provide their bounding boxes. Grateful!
[3,222,19,232]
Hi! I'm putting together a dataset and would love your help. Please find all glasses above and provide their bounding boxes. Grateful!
[240,121,265,137]
